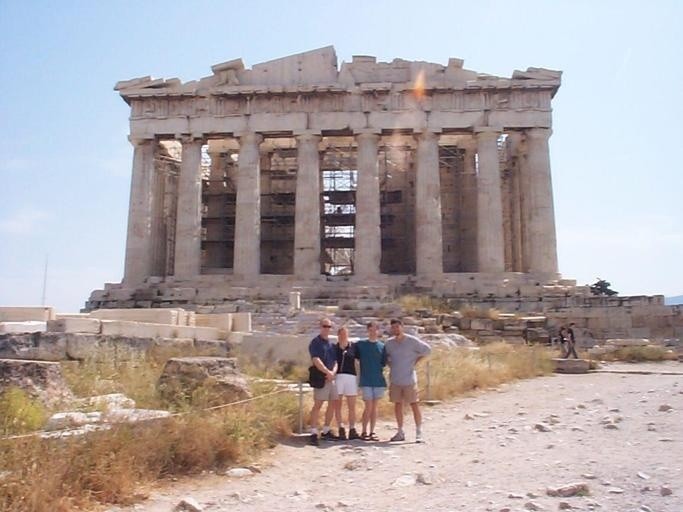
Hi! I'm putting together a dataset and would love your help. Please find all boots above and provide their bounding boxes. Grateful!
[349,428,359,439]
[339,428,346,440]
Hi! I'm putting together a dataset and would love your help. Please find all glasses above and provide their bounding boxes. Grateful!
[323,325,332,328]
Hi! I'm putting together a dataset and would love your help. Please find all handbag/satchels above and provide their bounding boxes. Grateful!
[309,367,325,388]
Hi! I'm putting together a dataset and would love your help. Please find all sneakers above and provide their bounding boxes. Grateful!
[392,433,405,441]
[416,436,422,443]
[310,434,319,446]
[321,430,339,441]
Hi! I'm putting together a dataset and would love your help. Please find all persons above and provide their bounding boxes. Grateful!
[308,319,339,445]
[356,321,387,441]
[332,326,360,441]
[383,318,431,442]
[562,322,579,359]
[559,326,568,354]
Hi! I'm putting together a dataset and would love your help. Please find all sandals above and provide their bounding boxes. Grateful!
[362,431,379,441]
